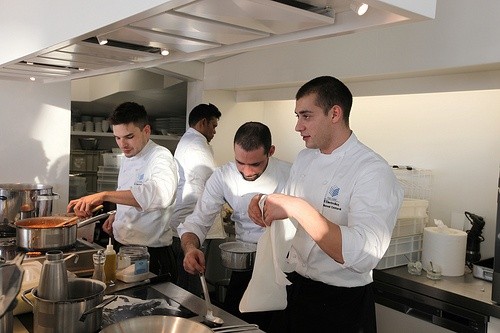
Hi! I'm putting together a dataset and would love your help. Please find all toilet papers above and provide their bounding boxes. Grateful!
[419,218,469,277]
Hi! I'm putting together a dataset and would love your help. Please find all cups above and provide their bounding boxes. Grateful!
[408,259,422,275]
[426,264,441,279]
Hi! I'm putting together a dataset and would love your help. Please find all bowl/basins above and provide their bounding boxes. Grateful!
[70,116,109,132]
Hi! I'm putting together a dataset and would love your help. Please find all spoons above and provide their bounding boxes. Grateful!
[199,271,224,327]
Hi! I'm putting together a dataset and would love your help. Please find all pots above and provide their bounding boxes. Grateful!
[79,136,100,150]
[14,210,117,250]
[21,278,118,333]
[0,182,60,228]
[219,242,257,270]
[98,315,258,333]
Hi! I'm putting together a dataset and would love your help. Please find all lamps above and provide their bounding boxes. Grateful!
[350,0,369,16]
[160,48,170,56]
[97,35,108,46]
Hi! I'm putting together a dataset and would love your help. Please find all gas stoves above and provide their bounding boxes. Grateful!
[0,236,100,262]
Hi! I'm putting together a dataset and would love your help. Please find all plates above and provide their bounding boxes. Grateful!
[155,117,186,134]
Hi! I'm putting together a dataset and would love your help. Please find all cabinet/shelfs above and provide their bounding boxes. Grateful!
[69,66,187,205]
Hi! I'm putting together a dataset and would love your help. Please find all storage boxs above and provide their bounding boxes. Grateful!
[374,234,423,269]
[390,200,432,235]
[472,257,494,281]
[71,149,110,192]
[96,165,120,192]
[392,166,434,200]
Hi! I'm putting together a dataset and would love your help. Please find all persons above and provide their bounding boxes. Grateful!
[66,102,179,278]
[248,75,404,333]
[173,102,227,299]
[176,121,294,333]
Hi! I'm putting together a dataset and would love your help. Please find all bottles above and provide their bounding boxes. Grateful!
[37,250,79,301]
[116,246,150,283]
[93,237,117,284]
